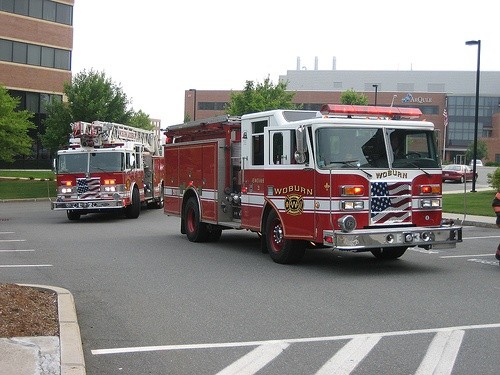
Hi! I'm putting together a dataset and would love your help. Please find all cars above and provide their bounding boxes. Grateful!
[471,159,483,167]
[442,164,478,183]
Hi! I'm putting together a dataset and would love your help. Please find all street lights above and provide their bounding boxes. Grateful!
[372,84,378,106]
[465,40,482,192]
[189,88,196,121]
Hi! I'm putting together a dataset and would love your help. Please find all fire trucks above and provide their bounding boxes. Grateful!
[160,105,464,266]
[49,120,162,220]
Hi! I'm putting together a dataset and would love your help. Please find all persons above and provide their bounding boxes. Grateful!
[493,186,500,261]
